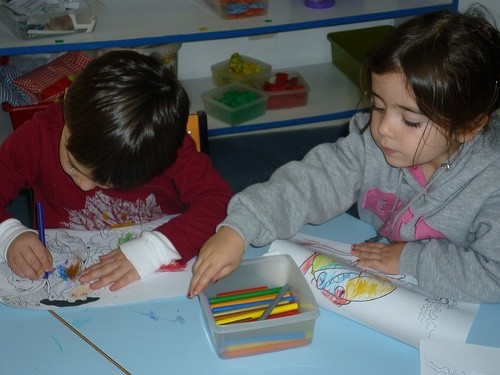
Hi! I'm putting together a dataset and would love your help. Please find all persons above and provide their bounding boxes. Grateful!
[0,49,233,291]
[186,2,500,305]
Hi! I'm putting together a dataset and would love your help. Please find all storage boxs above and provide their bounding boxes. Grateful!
[194,0,270,19]
[200,254,321,359]
[326,23,396,94]
[0,0,108,42]
[200,57,310,125]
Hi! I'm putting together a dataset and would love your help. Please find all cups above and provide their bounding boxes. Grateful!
[304,0,336,8]
[137,43,183,81]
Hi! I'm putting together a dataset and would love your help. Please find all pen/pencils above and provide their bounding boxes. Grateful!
[37,202,48,278]
[208,283,313,359]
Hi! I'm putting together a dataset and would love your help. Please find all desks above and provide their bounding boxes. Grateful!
[1,198,500,375]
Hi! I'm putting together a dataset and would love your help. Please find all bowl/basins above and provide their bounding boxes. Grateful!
[258,70,310,108]
[327,25,396,87]
[0,1,106,40]
[201,82,271,126]
[211,54,272,90]
[198,254,319,358]
[208,0,269,20]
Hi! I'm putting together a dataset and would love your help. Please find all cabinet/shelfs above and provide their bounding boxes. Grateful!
[0,0,460,142]
